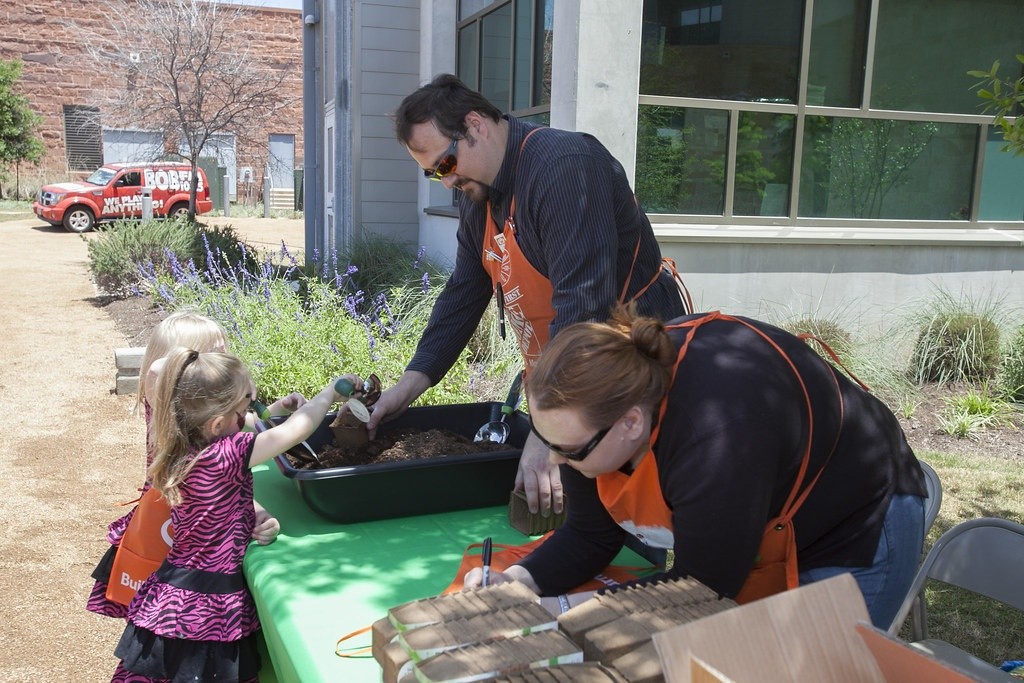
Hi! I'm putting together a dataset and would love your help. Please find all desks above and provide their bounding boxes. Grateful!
[240,457,658,683]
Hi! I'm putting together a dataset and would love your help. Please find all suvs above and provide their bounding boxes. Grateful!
[31,160,214,235]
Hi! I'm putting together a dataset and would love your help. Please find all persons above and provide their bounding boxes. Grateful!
[85,308,313,618]
[109,348,365,683]
[328,73,691,517]
[464,300,931,632]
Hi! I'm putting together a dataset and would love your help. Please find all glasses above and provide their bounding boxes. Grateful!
[423,131,459,182]
[529,415,614,461]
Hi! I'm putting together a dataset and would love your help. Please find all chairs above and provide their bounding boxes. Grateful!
[911,460,942,642]
[885,518,1024,683]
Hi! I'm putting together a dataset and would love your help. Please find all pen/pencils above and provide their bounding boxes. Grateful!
[481,536,492,588]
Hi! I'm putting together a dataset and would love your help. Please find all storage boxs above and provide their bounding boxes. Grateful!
[653,572,986,683]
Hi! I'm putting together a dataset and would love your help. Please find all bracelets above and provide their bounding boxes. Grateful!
[558,595,571,614]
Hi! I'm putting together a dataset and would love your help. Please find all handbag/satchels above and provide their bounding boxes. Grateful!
[106,488,174,605]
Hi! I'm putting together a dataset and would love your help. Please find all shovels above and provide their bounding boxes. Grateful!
[334,374,382,408]
[248,398,318,461]
[472,368,528,444]
